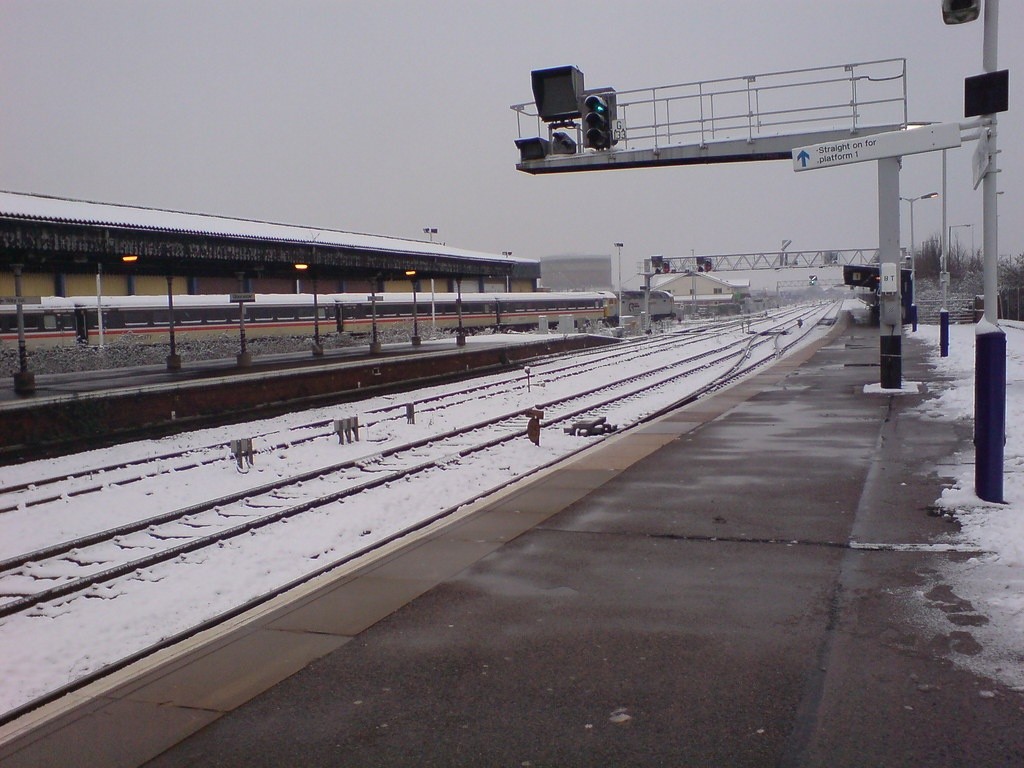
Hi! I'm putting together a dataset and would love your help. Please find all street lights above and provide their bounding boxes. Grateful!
[899,191,940,333]
[421,226,438,328]
[995,191,1005,273]
[940,0,1008,505]
[947,224,972,271]
[921,239,939,265]
[501,250,513,292]
[614,241,623,317]
[900,120,950,358]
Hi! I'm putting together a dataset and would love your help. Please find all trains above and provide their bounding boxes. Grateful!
[0,286,621,352]
[611,289,677,322]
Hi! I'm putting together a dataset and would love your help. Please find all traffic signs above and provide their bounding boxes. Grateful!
[789,120,962,173]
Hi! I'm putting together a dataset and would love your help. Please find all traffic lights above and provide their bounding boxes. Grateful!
[697,266,703,272]
[579,87,617,151]
[550,131,578,155]
[514,136,549,160]
[531,66,586,123]
[696,256,704,265]
[703,261,711,272]
[651,255,662,267]
[655,268,661,274]
[661,261,670,274]
